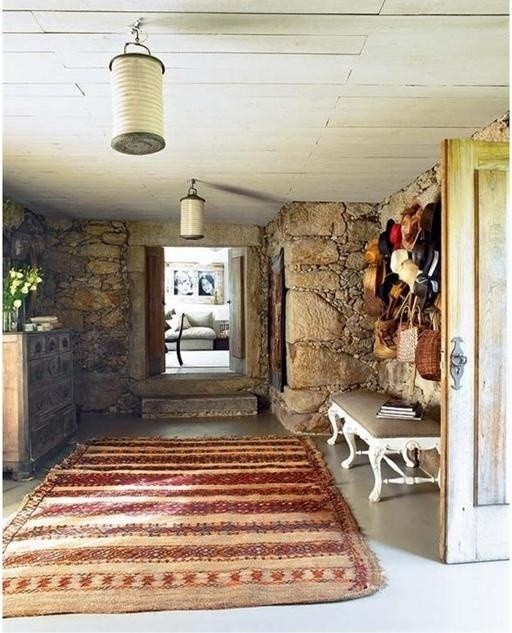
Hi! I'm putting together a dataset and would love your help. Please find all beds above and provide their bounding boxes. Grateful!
[163,310,216,350]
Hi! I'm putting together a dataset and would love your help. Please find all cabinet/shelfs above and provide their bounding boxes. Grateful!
[2,326,78,481]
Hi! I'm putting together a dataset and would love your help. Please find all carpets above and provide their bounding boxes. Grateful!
[2,434,380,618]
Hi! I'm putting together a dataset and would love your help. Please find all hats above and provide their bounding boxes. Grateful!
[363,202,440,314]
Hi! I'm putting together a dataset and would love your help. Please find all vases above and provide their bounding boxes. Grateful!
[2,305,17,332]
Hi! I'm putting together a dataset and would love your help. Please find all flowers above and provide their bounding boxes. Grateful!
[3,264,43,313]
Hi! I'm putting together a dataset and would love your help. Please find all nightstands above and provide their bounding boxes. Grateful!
[214,337,229,349]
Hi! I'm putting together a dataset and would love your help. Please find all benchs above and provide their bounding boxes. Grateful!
[327,390,444,502]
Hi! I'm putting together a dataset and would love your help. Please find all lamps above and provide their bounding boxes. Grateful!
[108,18,165,154]
[178,178,206,239]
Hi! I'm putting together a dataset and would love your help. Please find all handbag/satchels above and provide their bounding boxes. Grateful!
[373,297,441,380]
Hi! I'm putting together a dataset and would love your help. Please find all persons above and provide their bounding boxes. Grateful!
[199,273,214,296]
[174,271,193,295]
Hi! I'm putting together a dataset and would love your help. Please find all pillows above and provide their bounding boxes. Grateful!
[165,311,214,330]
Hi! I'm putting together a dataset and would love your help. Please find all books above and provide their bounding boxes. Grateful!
[376,397,424,421]
